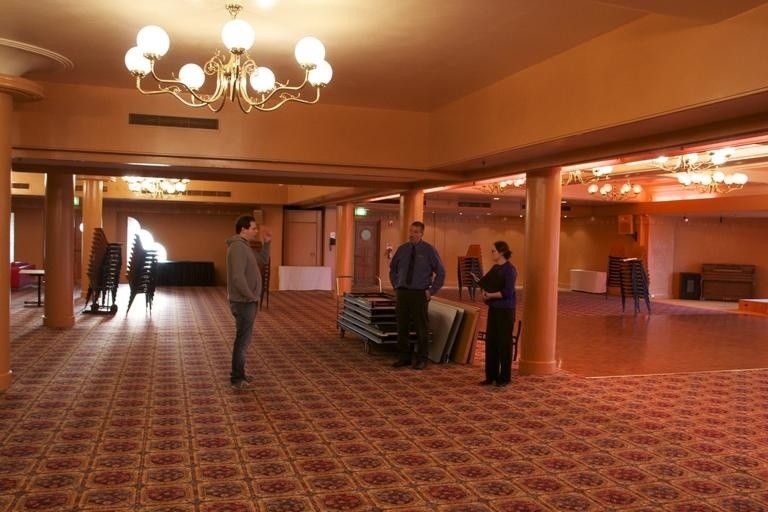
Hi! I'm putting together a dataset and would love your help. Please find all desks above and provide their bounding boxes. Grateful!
[570,269,608,294]
[18,269,46,308]
[153,261,217,286]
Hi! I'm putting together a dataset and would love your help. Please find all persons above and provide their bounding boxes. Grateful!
[467,240,519,387]
[225,213,272,393]
[388,221,445,371]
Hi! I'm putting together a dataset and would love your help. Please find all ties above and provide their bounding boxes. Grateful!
[404,246,418,287]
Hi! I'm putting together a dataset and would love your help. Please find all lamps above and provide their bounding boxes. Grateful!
[483,146,749,196]
[124,4,333,115]
[122,173,191,202]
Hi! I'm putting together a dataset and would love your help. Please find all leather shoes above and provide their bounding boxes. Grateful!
[413,360,427,370]
[392,360,413,368]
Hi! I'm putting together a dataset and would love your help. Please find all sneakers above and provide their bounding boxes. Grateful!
[230,378,259,392]
[245,375,257,384]
[495,377,511,387]
[480,378,496,385]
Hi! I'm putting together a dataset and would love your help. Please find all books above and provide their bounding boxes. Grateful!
[468,265,503,292]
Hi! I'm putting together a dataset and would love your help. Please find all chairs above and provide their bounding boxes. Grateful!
[83,227,122,315]
[605,255,626,298]
[124,234,160,314]
[256,256,272,309]
[619,257,652,316]
[457,244,486,303]
[477,318,522,361]
[11,261,36,292]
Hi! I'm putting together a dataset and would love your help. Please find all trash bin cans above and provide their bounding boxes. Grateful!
[680,272,702,300]
